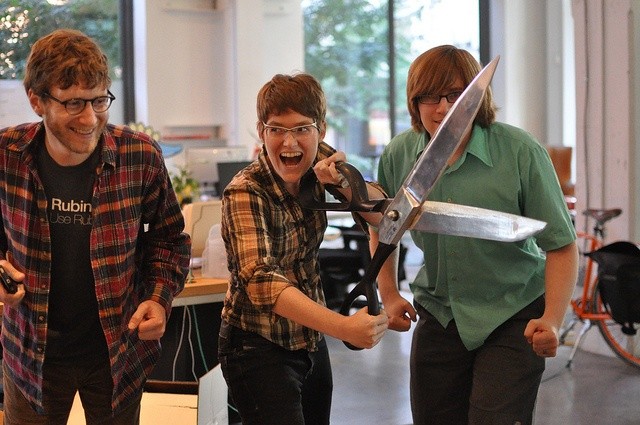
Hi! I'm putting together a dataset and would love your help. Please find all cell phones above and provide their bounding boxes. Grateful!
[0,265,18,295]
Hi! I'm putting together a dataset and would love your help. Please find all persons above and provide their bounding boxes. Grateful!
[217,70,385,425]
[366,44,578,424]
[0,29,192,424]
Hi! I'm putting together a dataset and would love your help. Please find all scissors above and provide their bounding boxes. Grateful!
[298,53,548,351]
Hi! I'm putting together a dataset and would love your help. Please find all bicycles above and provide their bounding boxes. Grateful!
[558,208,639,368]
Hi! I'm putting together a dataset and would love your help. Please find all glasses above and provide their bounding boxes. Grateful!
[42,88,116,115]
[420,91,463,105]
[261,120,319,139]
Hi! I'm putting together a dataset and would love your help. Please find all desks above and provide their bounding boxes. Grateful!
[144,265,229,393]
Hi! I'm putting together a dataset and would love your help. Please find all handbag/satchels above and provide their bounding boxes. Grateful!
[582,242,639,325]
